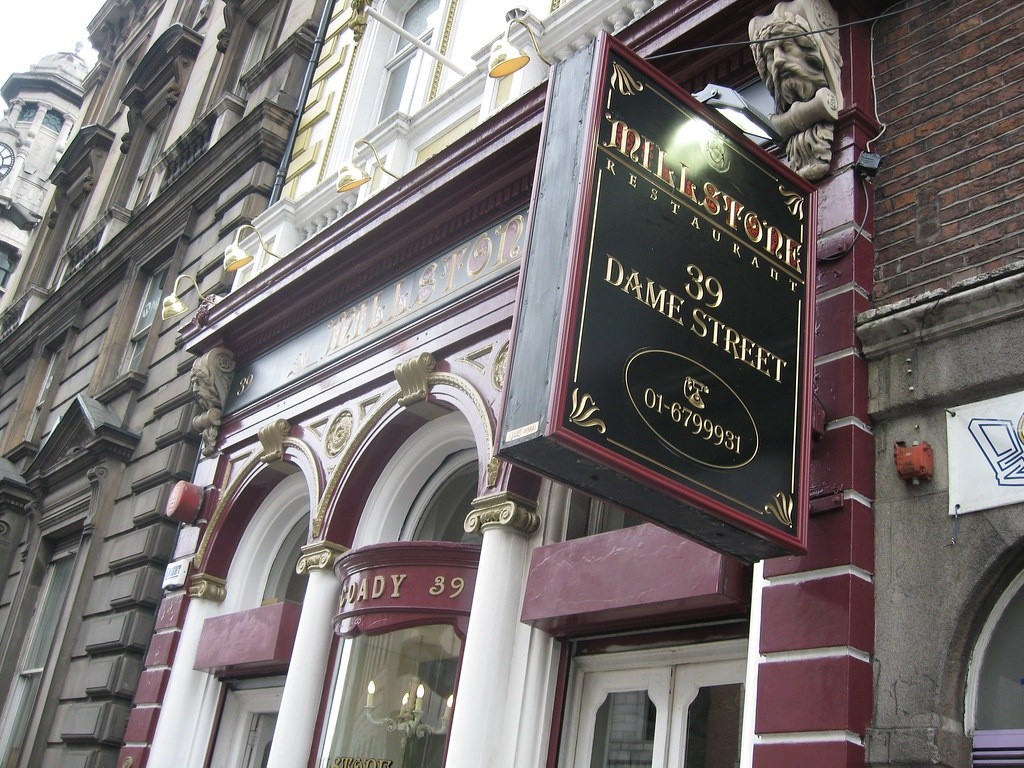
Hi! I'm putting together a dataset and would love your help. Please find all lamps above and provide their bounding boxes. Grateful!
[223,222,283,273]
[367,637,454,748]
[335,140,400,193]
[162,274,208,321]
[488,19,553,78]
